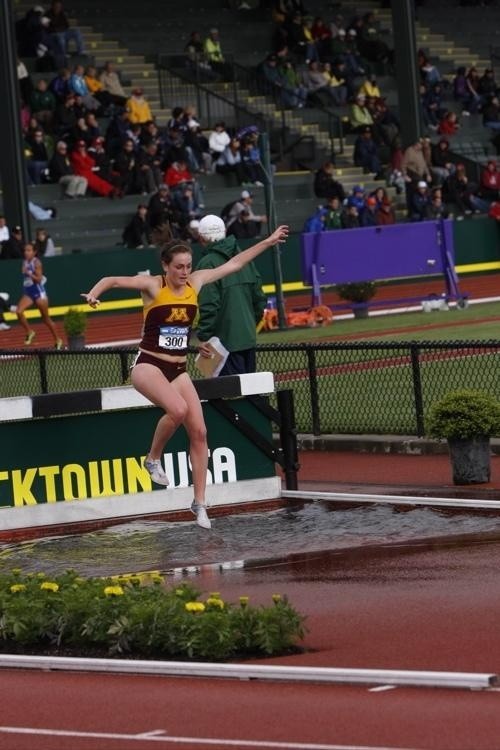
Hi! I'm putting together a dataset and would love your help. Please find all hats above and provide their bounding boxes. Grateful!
[353,187,366,191]
[417,181,427,187]
[242,190,256,200]
[368,198,376,206]
[190,214,226,243]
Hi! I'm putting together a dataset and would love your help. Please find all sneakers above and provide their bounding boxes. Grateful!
[144,453,170,486]
[25,331,36,346]
[190,499,211,529]
[55,339,63,349]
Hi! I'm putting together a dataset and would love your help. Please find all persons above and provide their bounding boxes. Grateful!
[2,2,500,258]
[190,215,267,377]
[79,224,290,530]
[1,244,64,351]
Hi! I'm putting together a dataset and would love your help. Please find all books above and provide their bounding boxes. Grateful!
[195,344,223,376]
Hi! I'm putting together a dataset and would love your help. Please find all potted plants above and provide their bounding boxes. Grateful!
[425,388,500,485]
